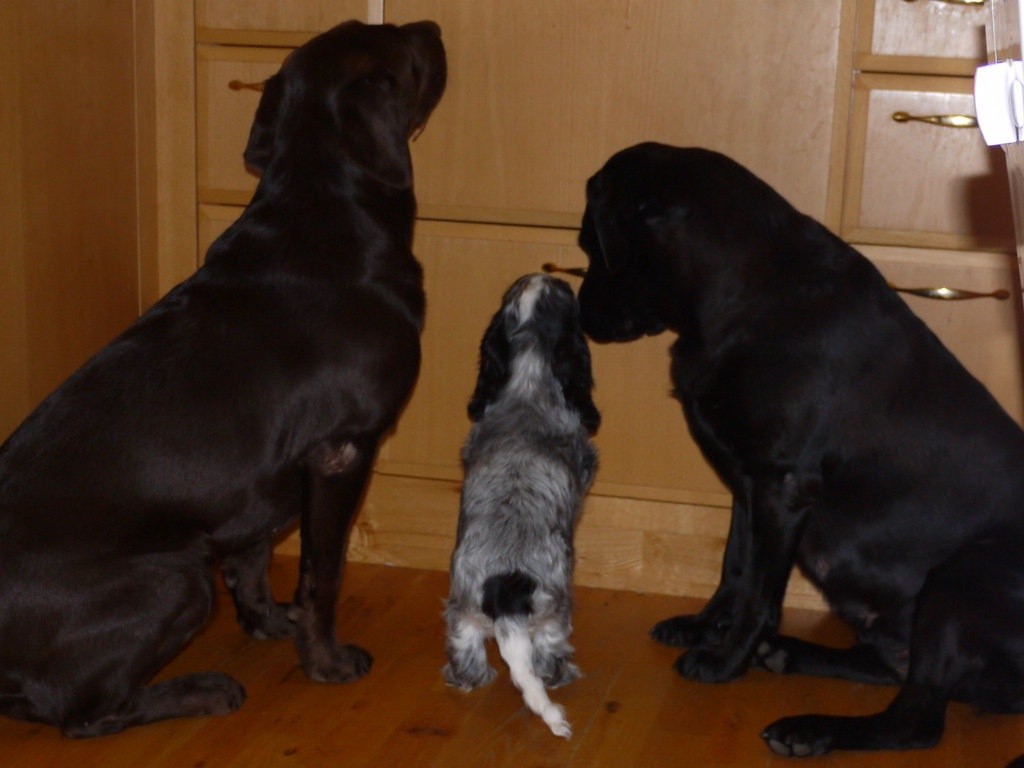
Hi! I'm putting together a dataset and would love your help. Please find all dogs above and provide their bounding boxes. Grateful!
[568,141,1024,768]
[444,271,603,740]
[0,19,449,740]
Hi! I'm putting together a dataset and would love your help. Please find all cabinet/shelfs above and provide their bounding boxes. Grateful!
[151,1,1023,612]
